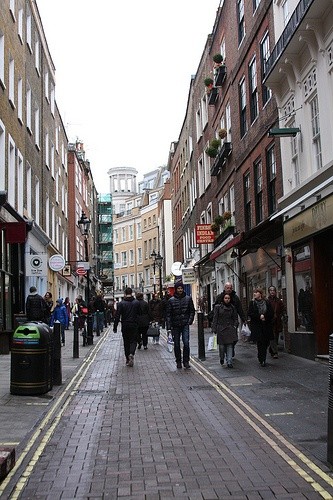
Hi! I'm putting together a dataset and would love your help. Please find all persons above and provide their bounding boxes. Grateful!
[134,293,151,350]
[210,294,239,368]
[113,288,142,366]
[25,283,312,369]
[247,287,274,367]
[167,283,195,368]
[267,287,284,358]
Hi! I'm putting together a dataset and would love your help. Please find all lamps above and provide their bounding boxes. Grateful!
[266,128,301,138]
[231,246,239,258]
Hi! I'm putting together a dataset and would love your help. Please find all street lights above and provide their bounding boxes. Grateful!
[155,250,165,329]
[77,209,92,345]
[150,249,157,293]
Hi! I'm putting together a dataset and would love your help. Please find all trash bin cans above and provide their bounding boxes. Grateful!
[10,321,53,396]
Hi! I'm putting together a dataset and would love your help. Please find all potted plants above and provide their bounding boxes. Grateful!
[213,222,235,248]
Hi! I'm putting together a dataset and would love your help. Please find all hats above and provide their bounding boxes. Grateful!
[30,287,36,292]
[56,298,63,304]
[175,283,184,290]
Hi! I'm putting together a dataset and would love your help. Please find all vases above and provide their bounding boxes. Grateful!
[214,66,225,86]
[207,88,218,105]
[209,141,231,176]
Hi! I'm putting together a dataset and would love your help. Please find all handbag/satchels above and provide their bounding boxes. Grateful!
[146,326,160,336]
[208,333,217,351]
[41,297,46,310]
[241,322,251,337]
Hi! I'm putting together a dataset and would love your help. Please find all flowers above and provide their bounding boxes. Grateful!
[214,64,222,70]
[206,84,213,95]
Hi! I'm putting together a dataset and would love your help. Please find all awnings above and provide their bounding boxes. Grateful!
[194,252,210,277]
[209,231,244,282]
[244,216,284,270]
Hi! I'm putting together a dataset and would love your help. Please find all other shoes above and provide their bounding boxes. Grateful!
[220,359,223,364]
[177,363,182,368]
[183,361,190,368]
[61,342,65,346]
[274,353,277,358]
[144,346,147,349]
[227,364,233,368]
[261,360,264,366]
[138,344,142,349]
[126,354,134,366]
